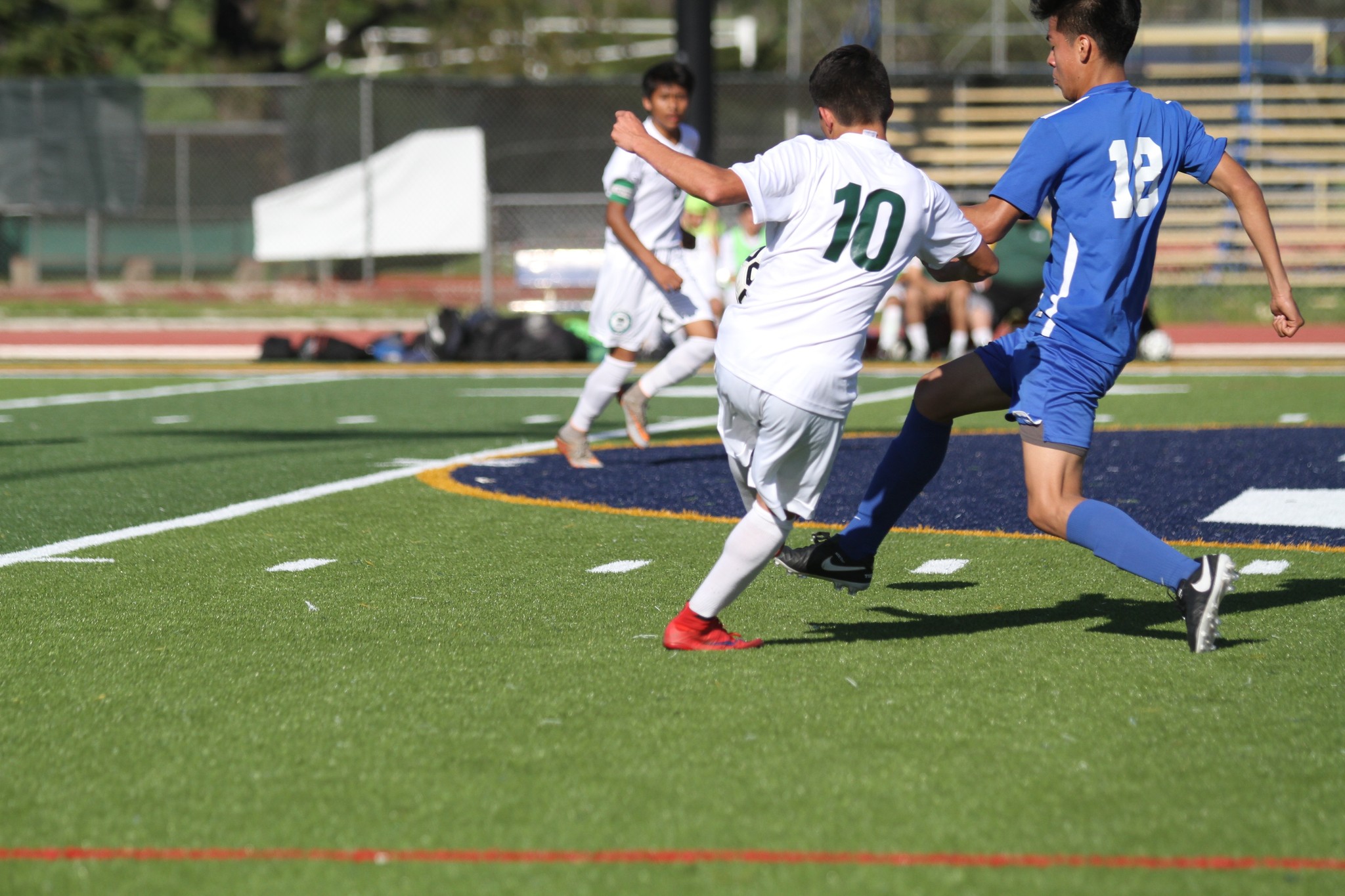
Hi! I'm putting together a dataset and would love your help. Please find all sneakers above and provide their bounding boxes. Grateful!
[1167,551,1239,653]
[662,615,763,651]
[616,383,651,451]
[774,534,874,597]
[555,429,604,469]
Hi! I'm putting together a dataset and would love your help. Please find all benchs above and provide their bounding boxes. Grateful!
[508,248,605,316]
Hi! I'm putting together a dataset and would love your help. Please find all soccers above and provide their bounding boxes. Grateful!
[1138,328,1173,363]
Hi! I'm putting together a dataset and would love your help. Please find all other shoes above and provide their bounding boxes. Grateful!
[909,341,930,362]
[878,340,908,363]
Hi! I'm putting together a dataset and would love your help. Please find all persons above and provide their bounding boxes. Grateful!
[554,61,766,469]
[772,0,1305,654]
[872,196,1172,363]
[609,44,1004,650]
